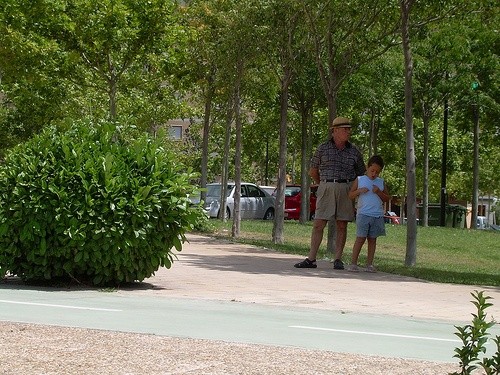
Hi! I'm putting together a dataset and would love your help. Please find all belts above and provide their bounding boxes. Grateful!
[320,178,352,183]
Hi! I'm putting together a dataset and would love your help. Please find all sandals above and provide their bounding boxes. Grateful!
[347,264,360,272]
[334,259,344,270]
[365,264,377,273]
[294,258,317,268]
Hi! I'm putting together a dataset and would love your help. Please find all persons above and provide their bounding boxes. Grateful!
[294,117,367,269]
[347,155,390,273]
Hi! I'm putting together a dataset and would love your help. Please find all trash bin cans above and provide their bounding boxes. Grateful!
[416,202,468,229]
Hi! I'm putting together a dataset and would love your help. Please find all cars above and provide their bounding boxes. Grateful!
[273,183,318,223]
[189,181,277,222]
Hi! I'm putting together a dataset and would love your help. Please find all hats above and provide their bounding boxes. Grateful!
[329,116,352,129]
